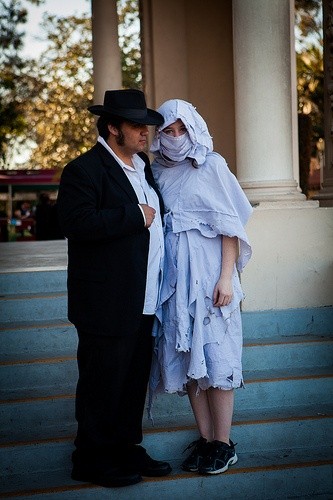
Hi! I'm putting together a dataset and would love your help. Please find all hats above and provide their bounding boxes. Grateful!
[88,89,165,125]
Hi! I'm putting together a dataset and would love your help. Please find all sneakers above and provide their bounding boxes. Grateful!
[181,436,211,471]
[201,438,238,474]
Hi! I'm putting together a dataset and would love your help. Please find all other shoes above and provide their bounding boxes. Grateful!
[123,445,172,477]
[71,449,142,488]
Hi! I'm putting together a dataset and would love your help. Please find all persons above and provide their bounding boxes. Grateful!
[56,89,173,487]
[33,193,66,241]
[148,98,253,476]
